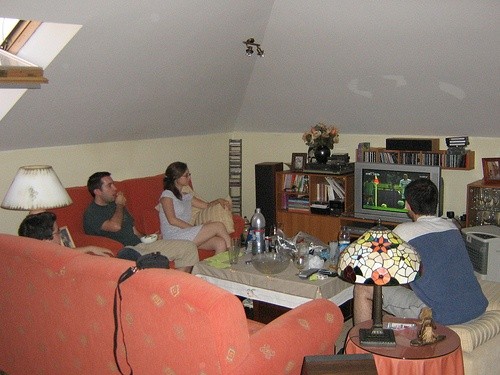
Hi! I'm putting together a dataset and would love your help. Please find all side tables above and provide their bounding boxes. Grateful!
[343,317,464,375]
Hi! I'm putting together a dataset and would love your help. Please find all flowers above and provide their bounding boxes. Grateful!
[301,122,340,149]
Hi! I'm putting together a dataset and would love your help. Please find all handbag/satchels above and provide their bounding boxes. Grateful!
[136,252,169,269]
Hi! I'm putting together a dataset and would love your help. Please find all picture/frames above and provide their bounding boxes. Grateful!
[59,225,76,250]
[292,153,307,172]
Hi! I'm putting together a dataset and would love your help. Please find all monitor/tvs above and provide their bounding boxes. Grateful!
[354,162,443,222]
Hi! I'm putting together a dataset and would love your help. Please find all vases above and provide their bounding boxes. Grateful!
[314,146,331,164]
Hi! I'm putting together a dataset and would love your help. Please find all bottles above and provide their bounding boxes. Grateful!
[241,208,272,256]
[338,226,350,253]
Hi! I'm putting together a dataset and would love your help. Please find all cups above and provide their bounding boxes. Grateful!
[227,238,242,264]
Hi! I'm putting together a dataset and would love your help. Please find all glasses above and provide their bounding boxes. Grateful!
[179,173,191,178]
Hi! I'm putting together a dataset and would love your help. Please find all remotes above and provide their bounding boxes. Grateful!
[298,268,319,280]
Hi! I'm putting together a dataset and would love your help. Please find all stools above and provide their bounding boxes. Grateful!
[445,279,500,375]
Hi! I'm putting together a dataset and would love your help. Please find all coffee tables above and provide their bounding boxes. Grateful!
[193,240,355,318]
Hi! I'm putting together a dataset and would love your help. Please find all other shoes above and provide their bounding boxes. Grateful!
[243,298,254,308]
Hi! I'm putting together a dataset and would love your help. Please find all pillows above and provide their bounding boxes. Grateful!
[155,184,234,234]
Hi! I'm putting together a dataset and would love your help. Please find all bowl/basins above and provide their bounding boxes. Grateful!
[251,252,290,274]
[140,233,158,243]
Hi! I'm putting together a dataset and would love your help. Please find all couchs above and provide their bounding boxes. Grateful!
[46,173,245,269]
[0,232,343,375]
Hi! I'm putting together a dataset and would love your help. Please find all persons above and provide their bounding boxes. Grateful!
[82,172,199,274]
[158,162,233,255]
[18,212,143,260]
[399,174,412,200]
[352,178,489,326]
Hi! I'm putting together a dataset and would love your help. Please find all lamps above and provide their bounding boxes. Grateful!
[242,38,265,58]
[1,165,74,214]
[336,218,423,347]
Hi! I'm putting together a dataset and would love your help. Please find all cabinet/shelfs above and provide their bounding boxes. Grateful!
[466,180,500,228]
[277,171,354,244]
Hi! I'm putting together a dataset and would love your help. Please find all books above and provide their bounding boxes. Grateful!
[357,142,466,168]
[282,173,346,214]
